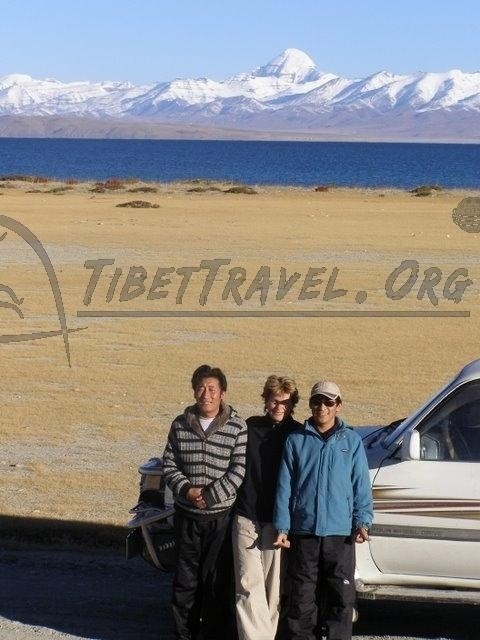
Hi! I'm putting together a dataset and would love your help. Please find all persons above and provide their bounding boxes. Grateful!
[272,381,375,640]
[230,375,305,640]
[161,364,249,640]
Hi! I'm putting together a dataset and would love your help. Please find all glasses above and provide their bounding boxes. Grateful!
[310,400,337,407]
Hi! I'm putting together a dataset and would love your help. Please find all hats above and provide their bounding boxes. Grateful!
[309,381,343,403]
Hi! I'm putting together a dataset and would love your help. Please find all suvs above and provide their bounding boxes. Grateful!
[126,358,480,623]
